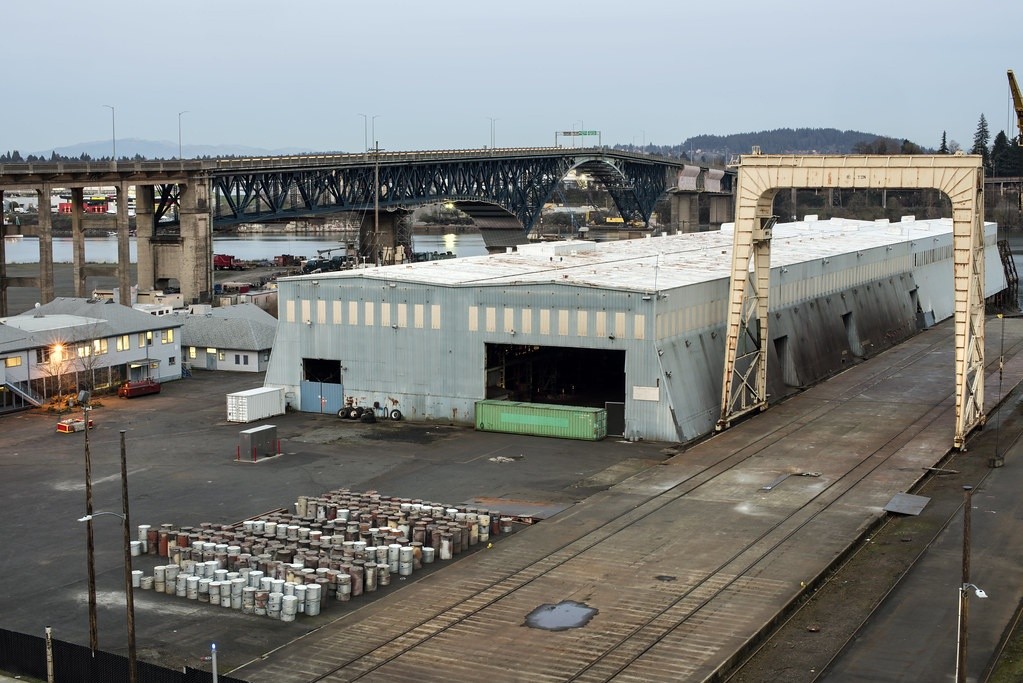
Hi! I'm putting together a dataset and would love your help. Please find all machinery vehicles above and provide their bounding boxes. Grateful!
[1005,69,1023,241]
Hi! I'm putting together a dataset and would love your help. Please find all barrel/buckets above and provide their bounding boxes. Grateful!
[130,490,513,622]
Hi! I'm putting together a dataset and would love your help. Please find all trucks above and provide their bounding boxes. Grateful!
[214,254,246,270]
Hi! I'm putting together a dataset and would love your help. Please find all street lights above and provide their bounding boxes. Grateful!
[955,584,988,683]
[106,105,116,160]
[179,111,187,161]
[362,114,367,153]
[77,390,97,648]
[55,344,62,422]
[373,115,378,149]
